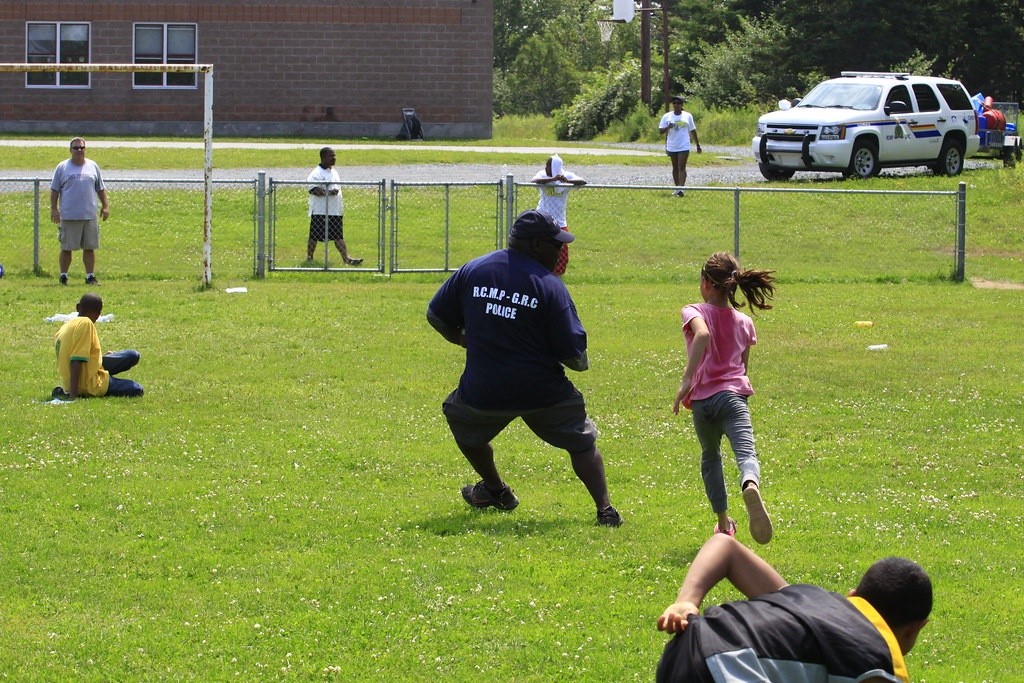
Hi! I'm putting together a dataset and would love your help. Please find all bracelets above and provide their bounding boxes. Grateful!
[696,143,700,146]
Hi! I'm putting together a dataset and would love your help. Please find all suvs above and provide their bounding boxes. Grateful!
[751,69,981,182]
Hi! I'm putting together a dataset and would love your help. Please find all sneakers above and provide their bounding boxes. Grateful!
[672,187,684,197]
[715,517,738,539]
[596,505,623,526]
[743,484,773,545]
[59,274,68,285]
[85,277,101,285]
[461,479,520,510]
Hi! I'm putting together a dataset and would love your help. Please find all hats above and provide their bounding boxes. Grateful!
[546,155,563,179]
[673,96,683,102]
[511,209,574,243]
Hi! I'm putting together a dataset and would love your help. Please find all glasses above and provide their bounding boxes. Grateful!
[71,146,84,150]
[672,101,682,105]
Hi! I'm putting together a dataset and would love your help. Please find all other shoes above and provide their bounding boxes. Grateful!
[345,258,364,266]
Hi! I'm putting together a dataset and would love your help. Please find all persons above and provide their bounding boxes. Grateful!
[674,252,782,544]
[422,210,625,527]
[528,153,587,275]
[50,136,110,286]
[658,96,702,197]
[53,293,146,397]
[304,147,364,266]
[652,532,936,683]
